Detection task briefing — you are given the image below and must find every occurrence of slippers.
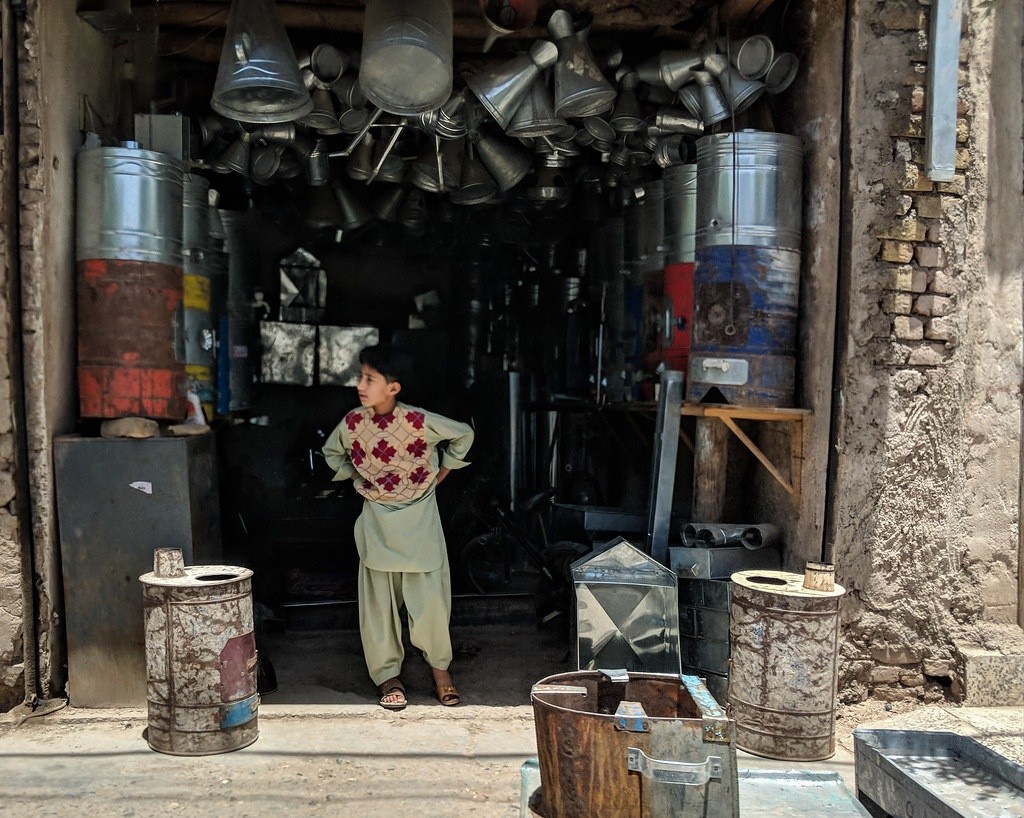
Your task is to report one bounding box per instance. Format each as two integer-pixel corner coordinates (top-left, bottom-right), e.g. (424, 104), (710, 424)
(433, 685), (460, 706)
(379, 678), (407, 708)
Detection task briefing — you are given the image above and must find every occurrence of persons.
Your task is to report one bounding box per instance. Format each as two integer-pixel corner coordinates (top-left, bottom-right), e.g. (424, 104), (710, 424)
(323, 344), (475, 708)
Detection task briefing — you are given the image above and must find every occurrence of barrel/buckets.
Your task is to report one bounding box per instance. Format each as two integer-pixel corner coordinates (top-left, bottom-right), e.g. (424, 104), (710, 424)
(73, 0), (803, 421)
(140, 547), (260, 755)
(728, 561), (845, 761)
